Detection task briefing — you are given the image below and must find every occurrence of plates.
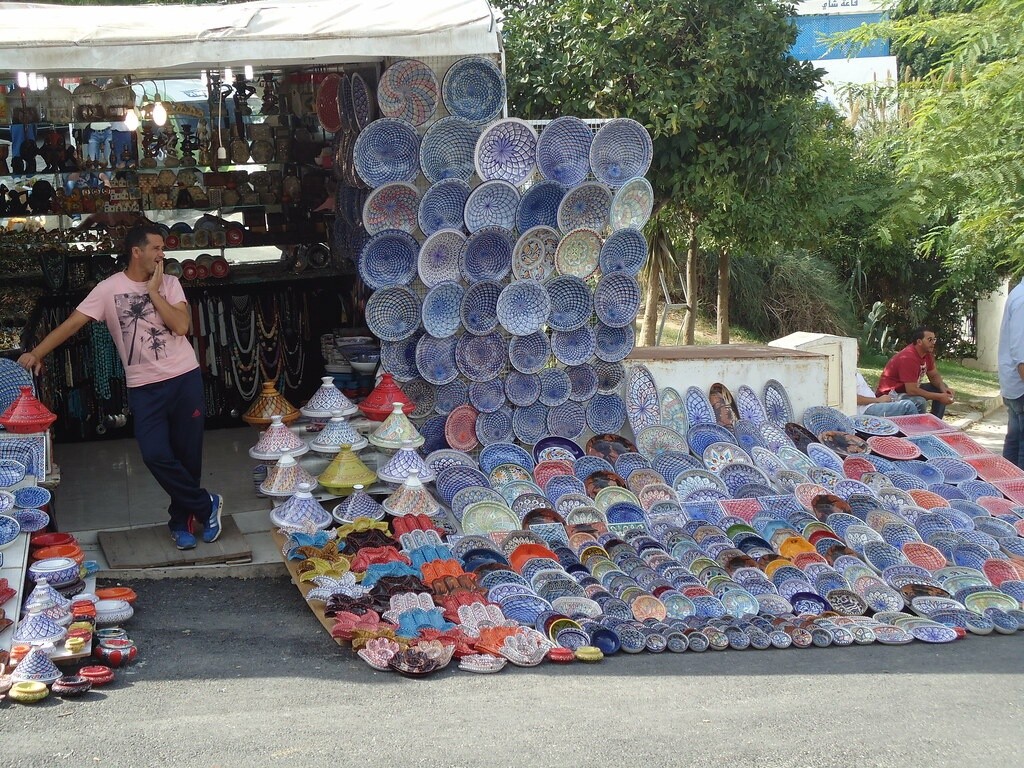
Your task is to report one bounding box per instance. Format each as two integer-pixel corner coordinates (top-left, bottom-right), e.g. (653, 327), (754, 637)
(0, 358), (49, 549)
(158, 216), (243, 281)
(314, 56), (1024, 661)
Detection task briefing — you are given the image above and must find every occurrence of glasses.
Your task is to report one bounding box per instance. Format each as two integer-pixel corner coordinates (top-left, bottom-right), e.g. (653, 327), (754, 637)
(922, 338), (938, 343)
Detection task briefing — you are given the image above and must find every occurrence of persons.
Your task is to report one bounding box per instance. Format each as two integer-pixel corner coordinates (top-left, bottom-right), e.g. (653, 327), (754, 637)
(17, 223), (224, 551)
(857, 344), (918, 415)
(876, 330), (954, 421)
(1000, 274), (1024, 473)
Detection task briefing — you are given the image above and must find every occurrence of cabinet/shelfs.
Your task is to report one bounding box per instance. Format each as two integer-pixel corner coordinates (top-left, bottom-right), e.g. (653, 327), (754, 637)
(0, 61), (382, 298)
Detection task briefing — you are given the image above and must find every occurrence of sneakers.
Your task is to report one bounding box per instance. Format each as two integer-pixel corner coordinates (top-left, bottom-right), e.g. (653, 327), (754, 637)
(171, 526), (196, 550)
(202, 494), (223, 543)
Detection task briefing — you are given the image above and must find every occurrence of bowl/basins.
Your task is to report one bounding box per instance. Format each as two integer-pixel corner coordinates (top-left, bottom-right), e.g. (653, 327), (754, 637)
(0, 534), (139, 702)
